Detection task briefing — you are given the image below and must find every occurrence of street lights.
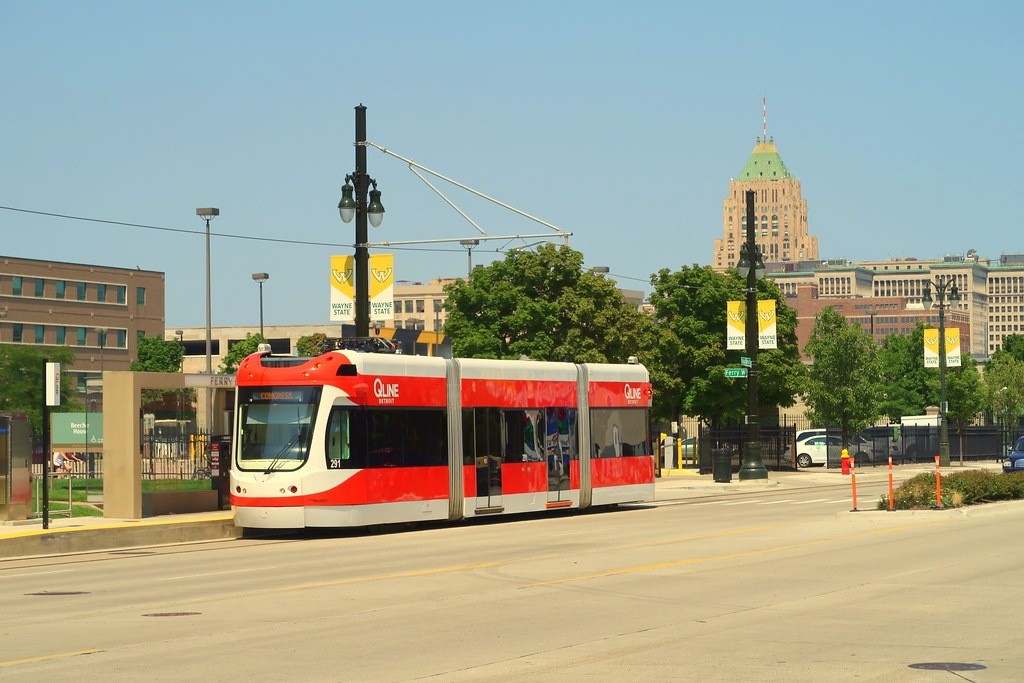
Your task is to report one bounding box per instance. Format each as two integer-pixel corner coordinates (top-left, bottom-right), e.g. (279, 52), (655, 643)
(338, 171), (386, 339)
(460, 239), (479, 286)
(736, 241), (768, 479)
(253, 273), (269, 343)
(923, 278), (960, 467)
(196, 207), (219, 442)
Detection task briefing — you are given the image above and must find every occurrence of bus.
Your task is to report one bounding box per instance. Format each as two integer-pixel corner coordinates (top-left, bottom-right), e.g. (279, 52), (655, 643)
(229, 344), (661, 536)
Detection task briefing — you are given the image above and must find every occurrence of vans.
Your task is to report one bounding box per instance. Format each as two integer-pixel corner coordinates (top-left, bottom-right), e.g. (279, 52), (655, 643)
(796, 429), (879, 454)
(858, 425), (935, 465)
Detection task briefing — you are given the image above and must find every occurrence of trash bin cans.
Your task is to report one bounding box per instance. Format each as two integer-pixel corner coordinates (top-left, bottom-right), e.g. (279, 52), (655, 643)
(711, 449), (732, 483)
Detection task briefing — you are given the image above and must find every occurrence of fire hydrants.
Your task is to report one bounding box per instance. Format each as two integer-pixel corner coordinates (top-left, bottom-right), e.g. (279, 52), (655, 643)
(841, 448), (850, 475)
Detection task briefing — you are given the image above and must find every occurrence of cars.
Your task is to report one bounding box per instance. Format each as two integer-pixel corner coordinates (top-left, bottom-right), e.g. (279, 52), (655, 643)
(681, 438), (698, 459)
(1003, 435), (1024, 472)
(783, 435), (875, 468)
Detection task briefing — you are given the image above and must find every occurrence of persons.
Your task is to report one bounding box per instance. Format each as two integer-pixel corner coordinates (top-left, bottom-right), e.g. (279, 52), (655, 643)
(53, 452), (81, 479)
